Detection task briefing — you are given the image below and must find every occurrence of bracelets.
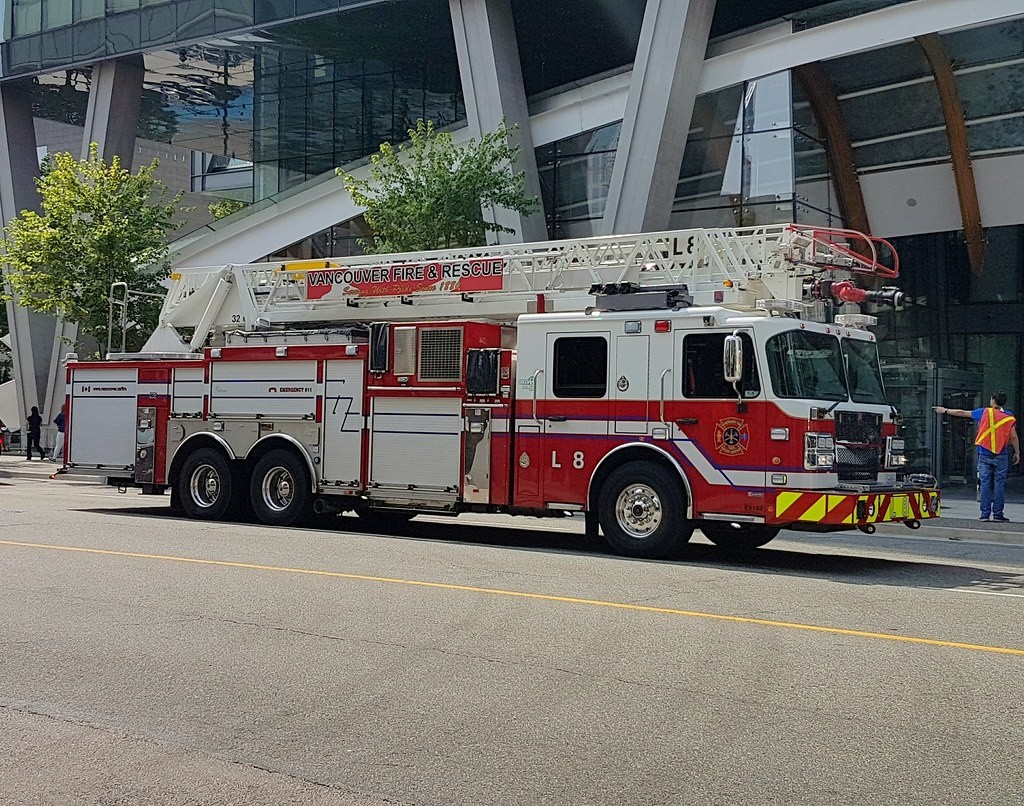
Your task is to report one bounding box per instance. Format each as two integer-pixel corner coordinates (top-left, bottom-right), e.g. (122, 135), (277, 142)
(945, 411), (947, 413)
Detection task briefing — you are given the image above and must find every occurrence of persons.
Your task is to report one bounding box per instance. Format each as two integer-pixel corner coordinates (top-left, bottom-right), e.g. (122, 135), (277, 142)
(24, 406), (45, 460)
(49, 403), (65, 462)
(931, 390), (1020, 523)
(0, 420), (12, 452)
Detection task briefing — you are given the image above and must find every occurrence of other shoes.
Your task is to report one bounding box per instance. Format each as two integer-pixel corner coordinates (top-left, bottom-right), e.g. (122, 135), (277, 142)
(49, 457), (56, 462)
(40, 454), (46, 460)
(26, 457), (31, 460)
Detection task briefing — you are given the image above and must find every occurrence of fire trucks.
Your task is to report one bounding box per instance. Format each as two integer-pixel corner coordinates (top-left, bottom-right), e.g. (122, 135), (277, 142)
(49, 224), (940, 559)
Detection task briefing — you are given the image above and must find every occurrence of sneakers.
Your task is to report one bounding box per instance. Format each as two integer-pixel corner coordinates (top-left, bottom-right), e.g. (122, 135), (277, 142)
(978, 515), (990, 521)
(993, 515), (1010, 522)
